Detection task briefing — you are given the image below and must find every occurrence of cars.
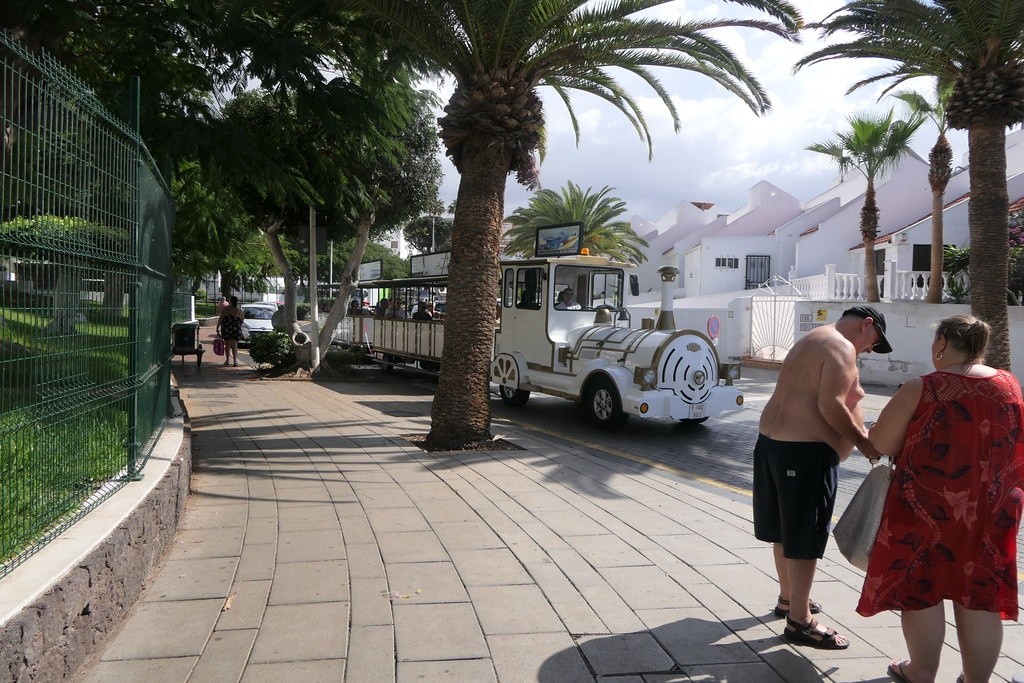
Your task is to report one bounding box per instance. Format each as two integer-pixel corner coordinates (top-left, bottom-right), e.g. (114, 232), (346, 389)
(236, 303), (279, 348)
(254, 300), (284, 314)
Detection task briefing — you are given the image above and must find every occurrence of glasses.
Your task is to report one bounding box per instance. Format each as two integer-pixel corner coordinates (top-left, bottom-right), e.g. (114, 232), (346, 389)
(873, 341), (881, 348)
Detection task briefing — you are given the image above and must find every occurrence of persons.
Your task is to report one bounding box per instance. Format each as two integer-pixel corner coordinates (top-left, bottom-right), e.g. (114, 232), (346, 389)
(411, 301), (440, 320)
(321, 303), (331, 311)
(216, 297), (230, 315)
(385, 298), (405, 319)
(854, 315), (1024, 683)
(555, 288), (581, 309)
(374, 298), (389, 318)
(272, 305), (286, 331)
(518, 291), (525, 307)
(216, 296), (245, 367)
(346, 300), (361, 317)
(752, 305), (893, 649)
(362, 299), (371, 315)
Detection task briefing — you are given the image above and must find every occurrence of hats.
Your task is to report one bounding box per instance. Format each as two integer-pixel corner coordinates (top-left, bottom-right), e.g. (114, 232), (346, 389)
(842, 305), (893, 353)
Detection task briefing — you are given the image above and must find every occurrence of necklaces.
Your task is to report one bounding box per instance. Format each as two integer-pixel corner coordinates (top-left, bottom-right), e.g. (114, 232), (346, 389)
(939, 362), (982, 370)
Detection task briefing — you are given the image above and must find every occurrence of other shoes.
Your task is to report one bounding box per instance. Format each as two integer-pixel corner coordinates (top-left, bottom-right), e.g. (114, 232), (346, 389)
(233, 363), (238, 366)
(224, 361), (229, 365)
(887, 658), (912, 683)
(956, 671), (964, 683)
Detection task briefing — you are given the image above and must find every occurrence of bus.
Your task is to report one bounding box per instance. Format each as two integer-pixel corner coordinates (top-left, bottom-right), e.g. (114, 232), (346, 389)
(317, 221), (746, 433)
(80, 278), (130, 308)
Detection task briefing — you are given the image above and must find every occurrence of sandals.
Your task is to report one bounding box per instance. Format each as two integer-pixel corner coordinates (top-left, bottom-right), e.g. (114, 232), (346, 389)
(784, 614), (850, 649)
(774, 596), (822, 617)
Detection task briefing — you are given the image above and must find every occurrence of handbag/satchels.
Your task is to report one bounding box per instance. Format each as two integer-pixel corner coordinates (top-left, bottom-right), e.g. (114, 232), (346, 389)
(214, 333), (224, 356)
(832, 454), (895, 573)
